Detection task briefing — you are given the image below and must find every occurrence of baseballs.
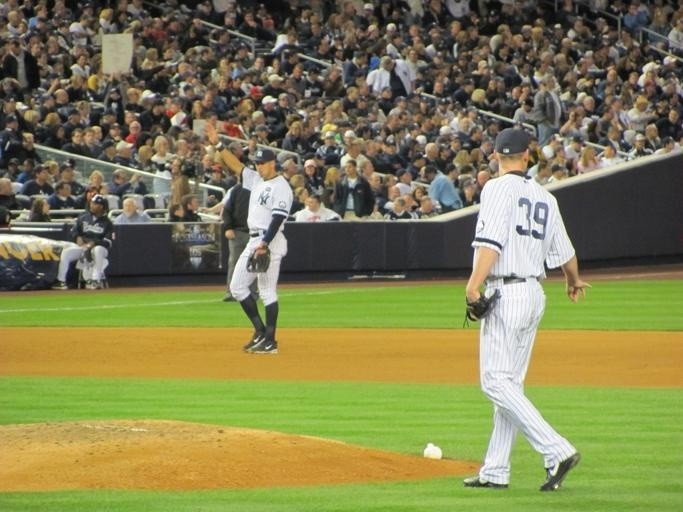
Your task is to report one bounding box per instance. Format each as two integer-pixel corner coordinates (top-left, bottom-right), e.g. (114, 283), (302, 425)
(485, 289), (495, 299)
(424, 446), (441, 459)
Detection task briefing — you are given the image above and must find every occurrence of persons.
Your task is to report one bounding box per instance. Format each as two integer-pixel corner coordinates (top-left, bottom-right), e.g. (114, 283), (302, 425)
(221, 182), (261, 304)
(462, 129), (593, 491)
(53, 194), (114, 290)
(204, 120), (293, 353)
(1, 1), (681, 229)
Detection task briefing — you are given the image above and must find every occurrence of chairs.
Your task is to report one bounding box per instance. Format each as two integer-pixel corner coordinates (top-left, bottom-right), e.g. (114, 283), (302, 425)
(0, 162), (172, 223)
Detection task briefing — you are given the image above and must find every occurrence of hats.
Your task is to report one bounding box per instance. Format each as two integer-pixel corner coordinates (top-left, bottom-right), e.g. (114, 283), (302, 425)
(2, 70), (83, 174)
(252, 148), (276, 163)
(90, 193), (106, 204)
(494, 127), (532, 155)
(141, 89), (156, 101)
(263, 66), (503, 169)
(140, 9), (152, 19)
(547, 56), (677, 143)
(363, 2), (397, 34)
(244, 161), (257, 171)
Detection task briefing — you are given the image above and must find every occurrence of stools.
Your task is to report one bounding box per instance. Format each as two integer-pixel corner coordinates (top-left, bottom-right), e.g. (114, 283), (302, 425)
(76, 255), (111, 291)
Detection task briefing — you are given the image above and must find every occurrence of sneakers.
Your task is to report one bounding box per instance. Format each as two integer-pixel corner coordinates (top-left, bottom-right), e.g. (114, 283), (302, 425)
(50, 280), (68, 290)
(242, 329), (266, 352)
(247, 339), (279, 355)
(222, 291), (237, 303)
(85, 279), (101, 290)
(537, 451), (581, 493)
(462, 476), (509, 490)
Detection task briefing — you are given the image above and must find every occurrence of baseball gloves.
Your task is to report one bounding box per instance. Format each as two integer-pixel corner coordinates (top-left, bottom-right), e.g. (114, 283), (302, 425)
(466, 290), (500, 321)
(247, 246), (270, 272)
